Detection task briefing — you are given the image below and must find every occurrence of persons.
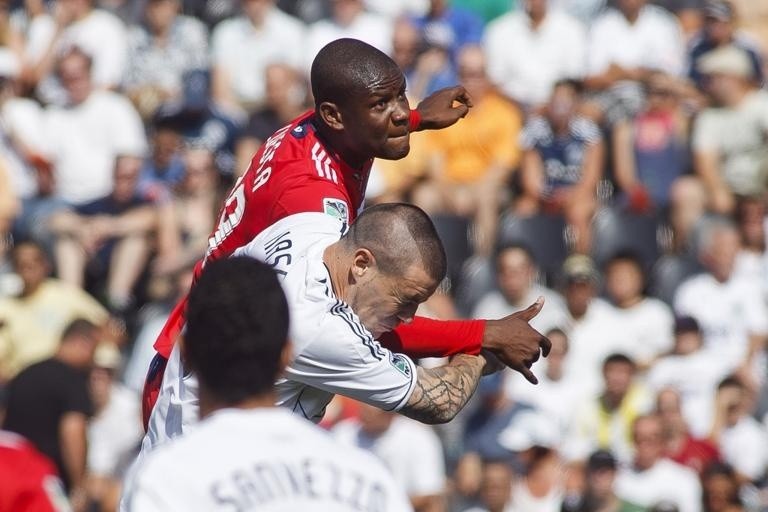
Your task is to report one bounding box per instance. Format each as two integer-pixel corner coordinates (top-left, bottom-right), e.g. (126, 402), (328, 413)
(2, 0), (768, 512)
